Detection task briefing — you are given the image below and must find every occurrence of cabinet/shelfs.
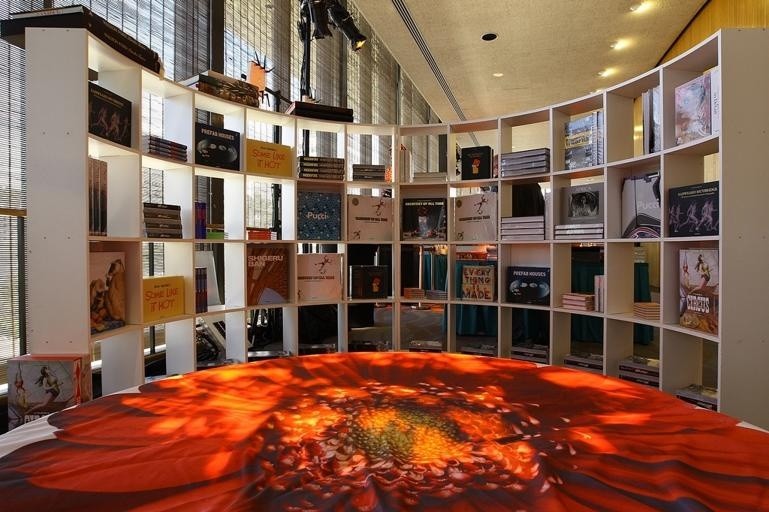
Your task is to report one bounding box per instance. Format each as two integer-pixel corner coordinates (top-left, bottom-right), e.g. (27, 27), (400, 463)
(24, 25), (769, 429)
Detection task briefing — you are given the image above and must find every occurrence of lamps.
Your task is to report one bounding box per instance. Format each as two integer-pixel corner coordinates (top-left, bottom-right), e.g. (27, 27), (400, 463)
(298, 0), (367, 103)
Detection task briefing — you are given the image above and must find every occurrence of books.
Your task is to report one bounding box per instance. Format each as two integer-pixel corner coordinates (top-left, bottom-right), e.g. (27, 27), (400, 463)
(347, 164), (392, 354)
(9, 4), (159, 63)
(89, 82), (132, 337)
(299, 155), (345, 356)
(139, 136), (185, 322)
(454, 146), (496, 357)
(555, 108), (604, 372)
(195, 108), (240, 371)
(1, 13), (160, 75)
(666, 65), (719, 410)
(500, 148), (550, 364)
(619, 86), (661, 387)
(399, 173), (447, 351)
(245, 138), (293, 363)
(178, 71), (354, 123)
